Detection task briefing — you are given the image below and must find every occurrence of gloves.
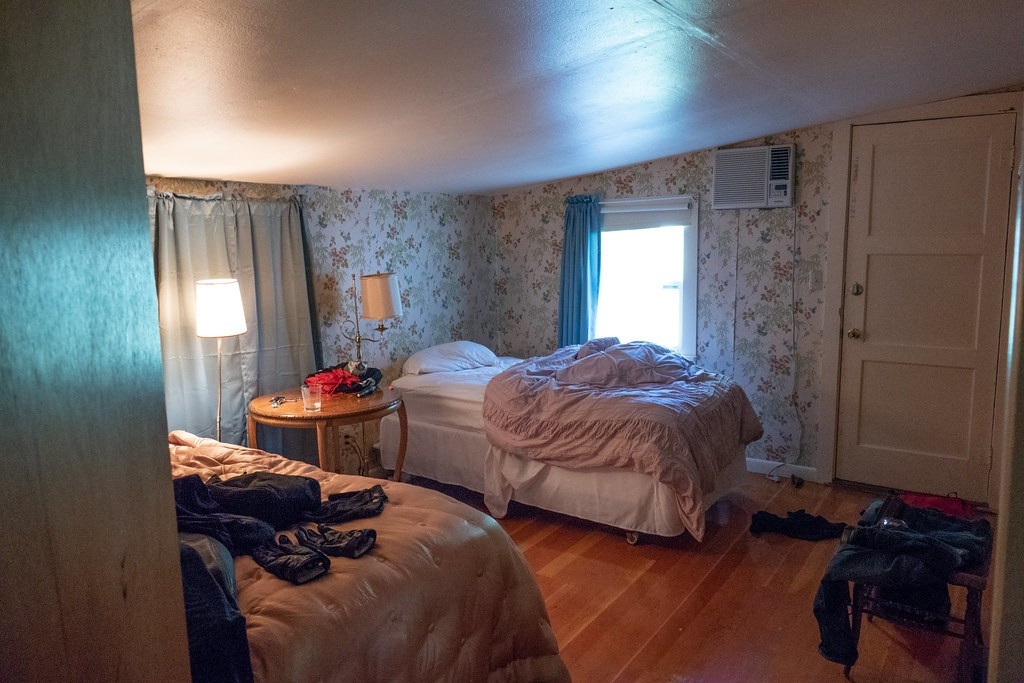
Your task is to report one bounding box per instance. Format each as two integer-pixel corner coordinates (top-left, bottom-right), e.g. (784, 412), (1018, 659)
(248, 532), (331, 586)
(304, 484), (388, 524)
(294, 523), (378, 562)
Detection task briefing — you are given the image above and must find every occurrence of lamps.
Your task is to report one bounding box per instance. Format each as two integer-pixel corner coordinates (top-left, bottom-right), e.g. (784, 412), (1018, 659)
(340, 273), (402, 476)
(195, 278), (248, 442)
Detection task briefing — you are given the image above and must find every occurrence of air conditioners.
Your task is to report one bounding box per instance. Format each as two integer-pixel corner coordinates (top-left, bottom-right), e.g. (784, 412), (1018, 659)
(712, 143), (794, 211)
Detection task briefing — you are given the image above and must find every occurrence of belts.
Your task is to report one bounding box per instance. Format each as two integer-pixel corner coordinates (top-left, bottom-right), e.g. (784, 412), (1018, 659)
(840, 495), (906, 544)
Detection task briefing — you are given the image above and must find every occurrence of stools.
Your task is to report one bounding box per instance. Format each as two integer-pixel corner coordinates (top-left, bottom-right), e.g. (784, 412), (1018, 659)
(843, 485), (988, 683)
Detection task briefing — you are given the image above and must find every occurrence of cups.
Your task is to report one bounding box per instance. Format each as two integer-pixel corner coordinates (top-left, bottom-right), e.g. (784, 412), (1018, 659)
(301, 384), (323, 412)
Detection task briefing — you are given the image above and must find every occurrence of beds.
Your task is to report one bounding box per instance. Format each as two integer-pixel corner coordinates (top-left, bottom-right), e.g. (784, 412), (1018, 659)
(168, 430), (572, 683)
(379, 355), (763, 545)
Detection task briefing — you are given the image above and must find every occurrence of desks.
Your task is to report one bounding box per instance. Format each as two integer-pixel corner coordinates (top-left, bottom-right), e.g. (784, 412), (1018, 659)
(248, 386), (409, 481)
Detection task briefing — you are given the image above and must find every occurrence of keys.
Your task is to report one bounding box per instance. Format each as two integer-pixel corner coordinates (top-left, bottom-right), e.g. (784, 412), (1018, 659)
(270, 396), (297, 407)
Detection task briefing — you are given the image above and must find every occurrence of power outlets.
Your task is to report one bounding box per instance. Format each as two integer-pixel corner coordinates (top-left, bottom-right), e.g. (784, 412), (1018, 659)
(342, 429), (351, 448)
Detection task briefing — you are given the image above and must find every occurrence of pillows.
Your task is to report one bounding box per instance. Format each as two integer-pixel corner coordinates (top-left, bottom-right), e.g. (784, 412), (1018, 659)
(401, 342), (503, 374)
